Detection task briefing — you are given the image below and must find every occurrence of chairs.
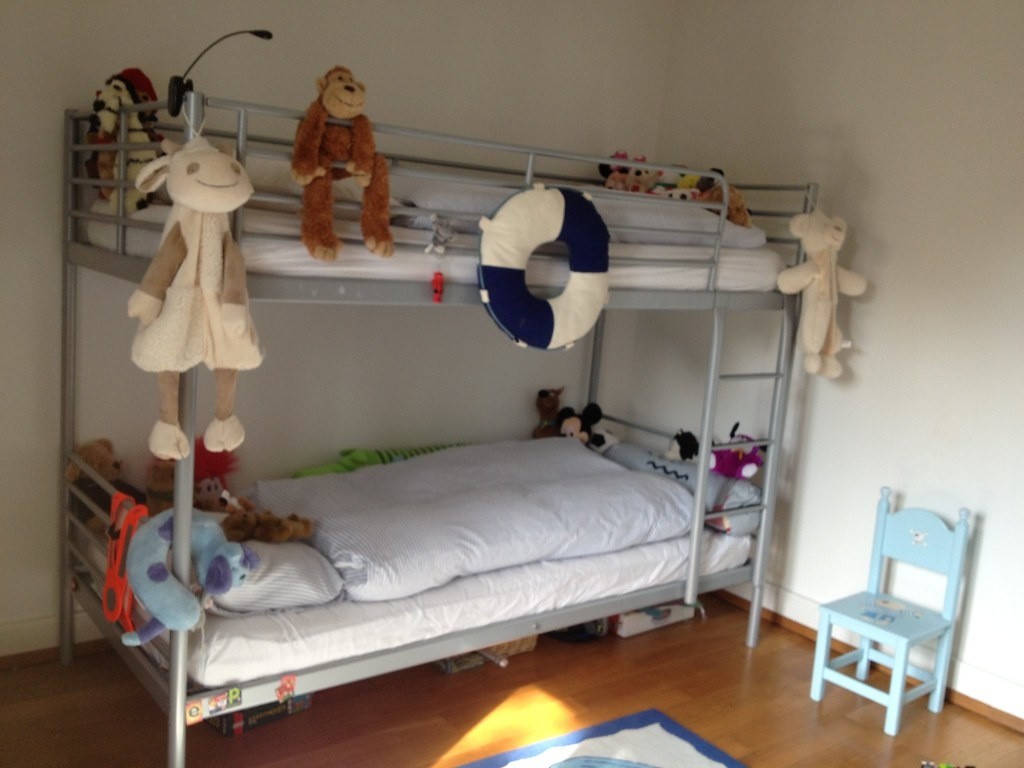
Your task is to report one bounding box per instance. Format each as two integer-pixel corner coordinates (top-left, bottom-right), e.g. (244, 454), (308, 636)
(808, 486), (970, 737)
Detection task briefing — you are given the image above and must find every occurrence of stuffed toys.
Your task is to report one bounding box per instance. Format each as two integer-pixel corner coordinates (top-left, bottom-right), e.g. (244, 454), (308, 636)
(776, 209), (867, 377)
(599, 148), (753, 227)
(664, 431), (717, 465)
(710, 422), (770, 479)
(143, 448), (176, 519)
(220, 499), (314, 542)
(532, 385), (565, 438)
(64, 438), (147, 538)
(121, 505), (260, 647)
(287, 65), (395, 261)
(124, 133), (263, 460)
(91, 68), (165, 216)
(289, 440), (497, 477)
(192, 433), (255, 513)
(556, 403), (602, 447)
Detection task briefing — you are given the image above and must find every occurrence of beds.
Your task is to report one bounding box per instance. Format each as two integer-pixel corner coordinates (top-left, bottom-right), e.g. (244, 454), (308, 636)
(57, 88), (821, 768)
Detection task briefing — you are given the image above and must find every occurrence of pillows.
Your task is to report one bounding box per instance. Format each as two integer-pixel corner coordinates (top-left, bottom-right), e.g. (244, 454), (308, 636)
(603, 442), (763, 536)
(198, 507), (345, 611)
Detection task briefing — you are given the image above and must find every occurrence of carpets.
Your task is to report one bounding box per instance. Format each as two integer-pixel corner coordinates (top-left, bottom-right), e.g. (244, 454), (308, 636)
(454, 709), (747, 768)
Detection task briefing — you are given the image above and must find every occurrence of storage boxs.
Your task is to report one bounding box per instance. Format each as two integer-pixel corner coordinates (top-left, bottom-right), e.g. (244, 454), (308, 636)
(608, 603), (695, 638)
(204, 694), (314, 738)
